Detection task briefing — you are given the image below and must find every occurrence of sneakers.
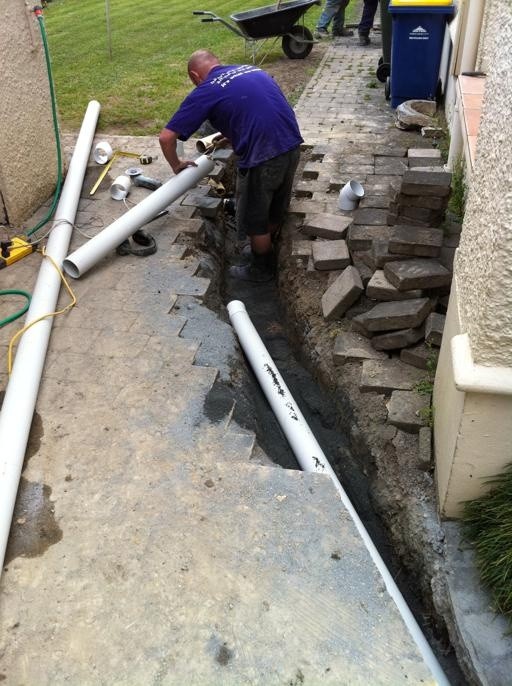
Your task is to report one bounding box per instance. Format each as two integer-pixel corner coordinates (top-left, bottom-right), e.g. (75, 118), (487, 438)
(230, 263), (273, 282)
(243, 244), (254, 260)
(314, 29), (330, 38)
(333, 29), (353, 36)
(359, 36), (371, 46)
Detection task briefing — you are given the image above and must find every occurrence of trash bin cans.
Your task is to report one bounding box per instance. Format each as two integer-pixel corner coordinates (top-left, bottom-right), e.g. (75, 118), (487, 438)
(376, 0), (455, 108)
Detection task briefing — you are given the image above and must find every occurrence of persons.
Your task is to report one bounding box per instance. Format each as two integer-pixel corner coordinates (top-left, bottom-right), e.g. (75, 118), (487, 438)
(357, 0), (380, 46)
(159, 50), (304, 281)
(314, 0), (353, 38)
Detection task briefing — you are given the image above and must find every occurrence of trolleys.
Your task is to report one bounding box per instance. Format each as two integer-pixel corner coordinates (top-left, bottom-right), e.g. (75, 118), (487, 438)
(192, 0), (322, 66)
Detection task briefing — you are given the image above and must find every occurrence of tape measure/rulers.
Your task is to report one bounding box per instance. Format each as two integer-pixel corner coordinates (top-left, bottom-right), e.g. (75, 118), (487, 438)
(89, 151), (152, 195)
